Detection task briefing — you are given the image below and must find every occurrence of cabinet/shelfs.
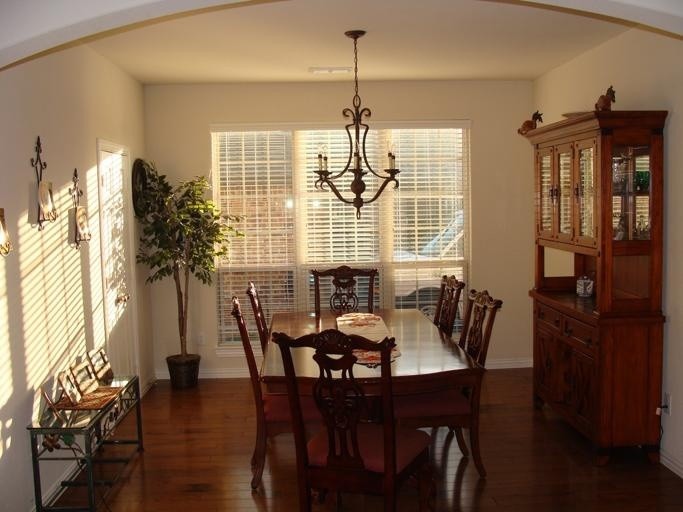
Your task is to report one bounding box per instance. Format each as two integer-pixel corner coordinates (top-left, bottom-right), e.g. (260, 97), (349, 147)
(520, 108), (670, 452)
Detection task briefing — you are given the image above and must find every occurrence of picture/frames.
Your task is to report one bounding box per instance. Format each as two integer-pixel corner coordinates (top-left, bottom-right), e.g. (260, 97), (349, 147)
(57, 370), (81, 405)
(88, 346), (112, 381)
(70, 360), (99, 395)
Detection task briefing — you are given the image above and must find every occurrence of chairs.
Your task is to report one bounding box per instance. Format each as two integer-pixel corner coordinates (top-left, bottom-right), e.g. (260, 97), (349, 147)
(432, 272), (465, 336)
(310, 263), (376, 311)
(395, 287), (503, 480)
(248, 280), (276, 358)
(231, 294), (337, 490)
(268, 326), (436, 511)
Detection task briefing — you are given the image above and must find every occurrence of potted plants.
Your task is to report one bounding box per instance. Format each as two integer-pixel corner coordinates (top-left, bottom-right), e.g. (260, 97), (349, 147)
(133, 158), (247, 388)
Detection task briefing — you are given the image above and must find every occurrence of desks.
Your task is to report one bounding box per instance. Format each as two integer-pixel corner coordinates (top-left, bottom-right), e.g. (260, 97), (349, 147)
(255, 308), (487, 512)
(25, 375), (148, 512)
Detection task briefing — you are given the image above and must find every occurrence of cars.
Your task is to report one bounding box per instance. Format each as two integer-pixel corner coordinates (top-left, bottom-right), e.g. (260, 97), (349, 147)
(421, 208), (465, 259)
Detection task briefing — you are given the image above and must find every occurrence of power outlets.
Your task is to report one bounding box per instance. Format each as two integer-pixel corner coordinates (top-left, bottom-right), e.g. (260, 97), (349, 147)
(663, 392), (671, 416)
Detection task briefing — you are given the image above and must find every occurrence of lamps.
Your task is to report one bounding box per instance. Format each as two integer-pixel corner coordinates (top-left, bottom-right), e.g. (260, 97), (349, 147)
(312, 28), (400, 220)
